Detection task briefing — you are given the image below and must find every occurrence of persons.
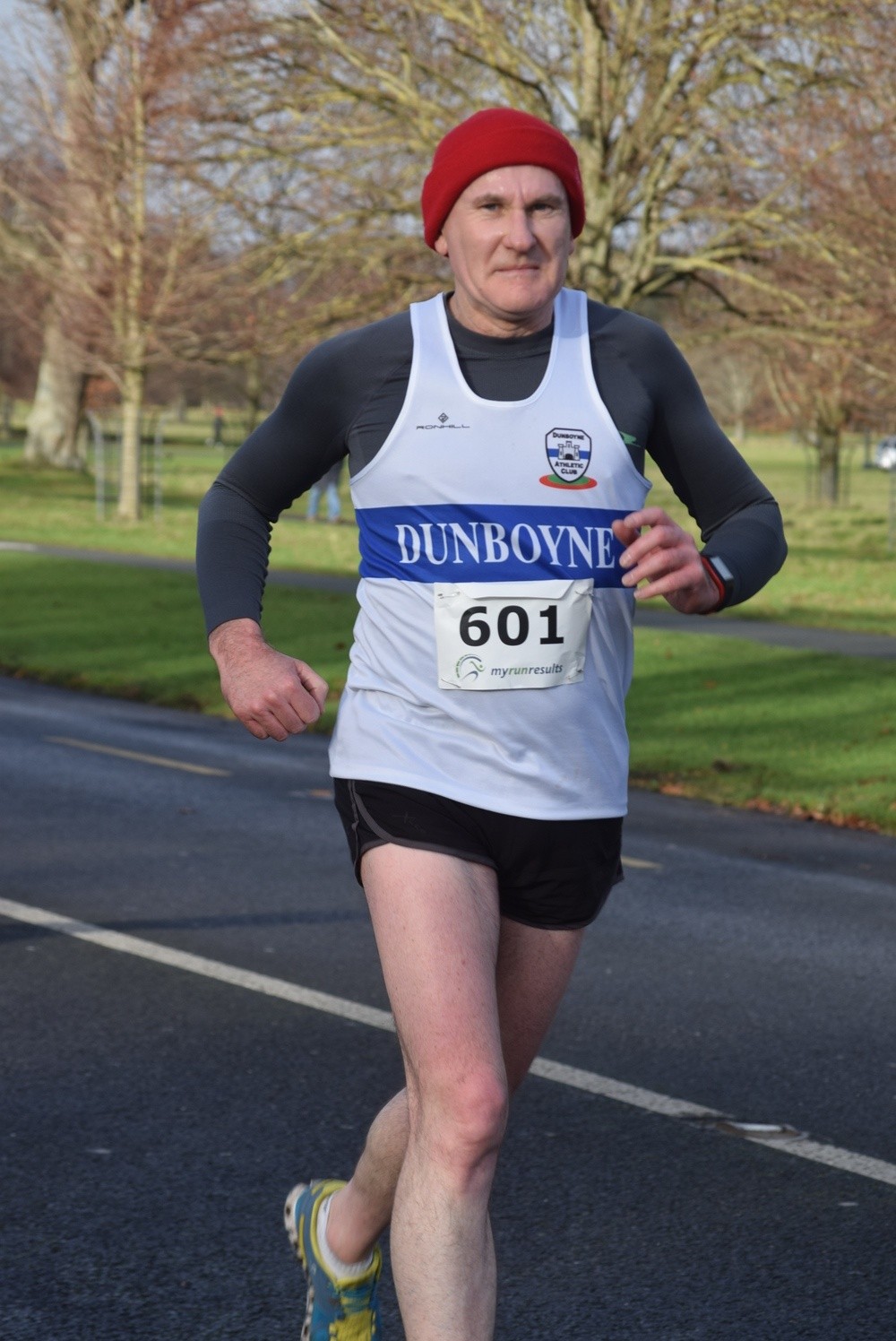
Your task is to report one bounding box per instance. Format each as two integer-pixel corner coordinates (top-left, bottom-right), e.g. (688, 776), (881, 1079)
(195, 106), (790, 1341)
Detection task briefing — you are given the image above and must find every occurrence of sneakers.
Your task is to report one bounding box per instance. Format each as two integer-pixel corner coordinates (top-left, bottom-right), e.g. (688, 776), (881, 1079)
(284, 1177), (383, 1341)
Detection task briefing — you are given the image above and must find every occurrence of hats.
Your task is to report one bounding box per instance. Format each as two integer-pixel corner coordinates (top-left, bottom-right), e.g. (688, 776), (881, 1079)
(421, 108), (586, 257)
